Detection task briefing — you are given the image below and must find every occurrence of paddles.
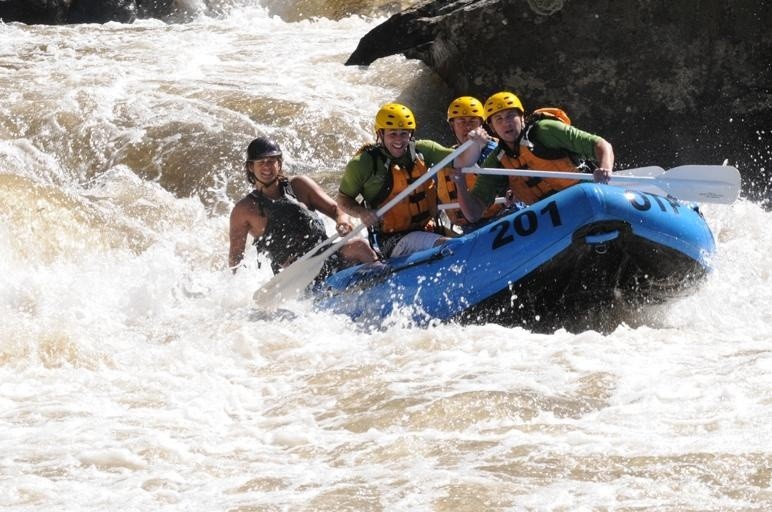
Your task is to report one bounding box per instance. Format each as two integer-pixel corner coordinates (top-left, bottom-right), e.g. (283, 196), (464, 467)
(461, 165), (741, 206)
(608, 167), (669, 202)
(252, 141), (472, 312)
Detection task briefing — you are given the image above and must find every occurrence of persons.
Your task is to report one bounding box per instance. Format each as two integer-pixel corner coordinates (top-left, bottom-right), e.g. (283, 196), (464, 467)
(333, 103), (489, 262)
(423, 94), (524, 234)
(227, 135), (380, 275)
(444, 90), (617, 226)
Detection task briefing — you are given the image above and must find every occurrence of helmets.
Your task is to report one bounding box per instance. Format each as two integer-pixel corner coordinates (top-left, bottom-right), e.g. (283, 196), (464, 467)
(375, 103), (416, 137)
(447, 96), (484, 123)
(247, 137), (282, 162)
(484, 91), (524, 122)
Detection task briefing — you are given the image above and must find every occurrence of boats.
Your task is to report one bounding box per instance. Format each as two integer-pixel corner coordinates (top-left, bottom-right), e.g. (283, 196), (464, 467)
(313, 182), (716, 335)
